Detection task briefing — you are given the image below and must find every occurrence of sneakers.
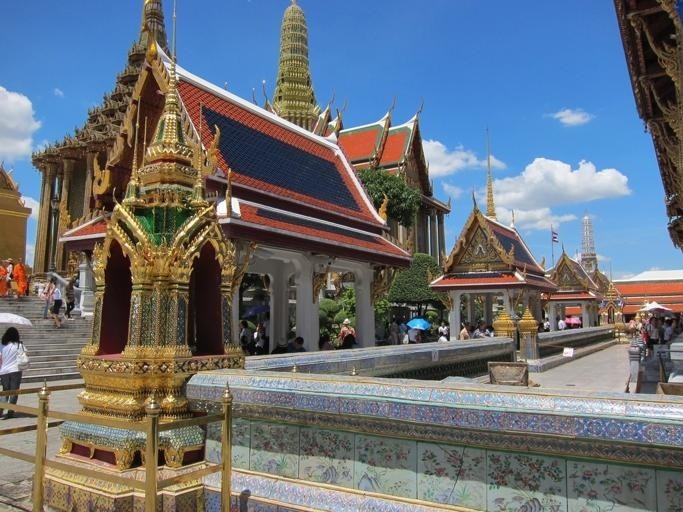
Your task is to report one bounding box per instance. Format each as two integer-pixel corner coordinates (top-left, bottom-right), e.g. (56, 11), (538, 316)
(62, 312), (76, 321)
(0, 413), (20, 420)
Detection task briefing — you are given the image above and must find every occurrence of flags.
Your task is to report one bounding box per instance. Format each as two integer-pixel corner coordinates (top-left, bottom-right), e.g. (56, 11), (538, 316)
(551, 230), (559, 242)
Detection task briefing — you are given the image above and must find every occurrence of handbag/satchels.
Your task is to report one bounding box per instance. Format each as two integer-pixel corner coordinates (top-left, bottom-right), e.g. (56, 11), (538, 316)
(16, 341), (30, 371)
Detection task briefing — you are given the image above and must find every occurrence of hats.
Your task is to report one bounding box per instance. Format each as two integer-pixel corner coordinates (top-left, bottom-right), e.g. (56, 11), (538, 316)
(343, 319), (351, 325)
(1, 257), (24, 263)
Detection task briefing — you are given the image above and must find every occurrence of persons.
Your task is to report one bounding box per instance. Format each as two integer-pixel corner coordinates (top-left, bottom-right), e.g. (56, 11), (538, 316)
(0, 326), (25, 419)
(0, 256), (78, 330)
